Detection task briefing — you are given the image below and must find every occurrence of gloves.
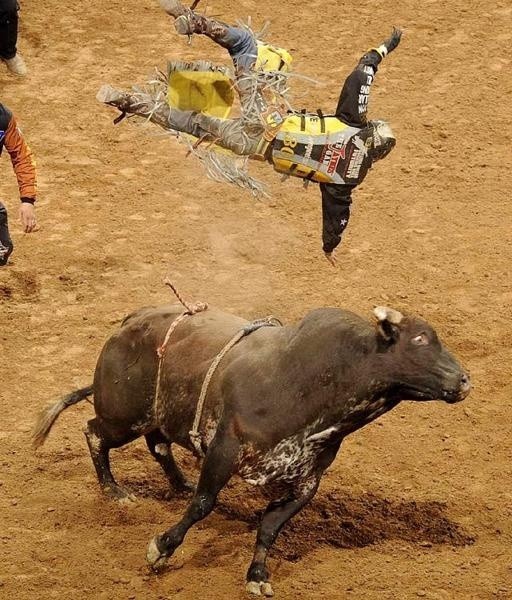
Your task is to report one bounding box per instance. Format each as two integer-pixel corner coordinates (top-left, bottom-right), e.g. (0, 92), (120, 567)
(380, 25), (403, 59)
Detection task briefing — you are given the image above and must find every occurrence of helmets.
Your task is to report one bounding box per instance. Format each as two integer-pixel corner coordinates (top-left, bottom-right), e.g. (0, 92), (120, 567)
(370, 120), (396, 162)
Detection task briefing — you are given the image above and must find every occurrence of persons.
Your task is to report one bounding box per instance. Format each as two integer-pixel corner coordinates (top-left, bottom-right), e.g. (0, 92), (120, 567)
(0, 0), (28, 77)
(96, 0), (404, 266)
(0, 102), (38, 266)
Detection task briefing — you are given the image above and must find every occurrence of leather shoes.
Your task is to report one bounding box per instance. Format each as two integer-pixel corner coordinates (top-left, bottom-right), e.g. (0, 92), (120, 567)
(95, 84), (124, 106)
(3, 51), (27, 75)
(162, 1), (195, 35)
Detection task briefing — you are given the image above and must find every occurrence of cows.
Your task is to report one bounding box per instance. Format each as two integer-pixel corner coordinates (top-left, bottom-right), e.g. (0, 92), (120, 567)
(29, 306), (472, 597)
